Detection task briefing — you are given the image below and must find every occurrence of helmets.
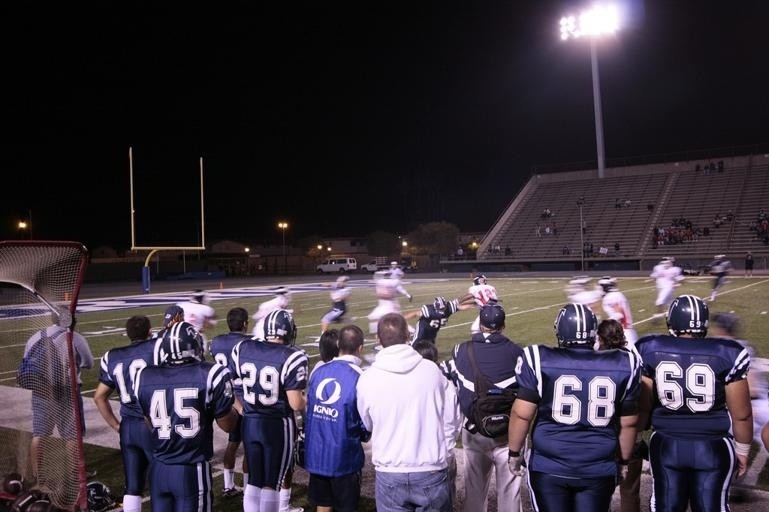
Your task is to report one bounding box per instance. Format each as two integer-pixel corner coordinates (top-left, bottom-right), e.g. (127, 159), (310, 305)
(552, 303), (598, 348)
(473, 275), (487, 285)
(665, 294), (709, 337)
(433, 295), (446, 315)
(264, 310), (297, 346)
(599, 276), (618, 292)
(164, 321), (203, 364)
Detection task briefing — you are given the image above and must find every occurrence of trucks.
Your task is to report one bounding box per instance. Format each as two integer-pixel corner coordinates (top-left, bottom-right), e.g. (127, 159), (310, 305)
(361, 257), (402, 275)
(316, 258), (357, 274)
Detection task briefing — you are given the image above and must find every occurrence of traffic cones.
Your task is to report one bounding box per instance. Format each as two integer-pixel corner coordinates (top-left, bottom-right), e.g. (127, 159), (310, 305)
(216, 280), (225, 289)
(62, 291), (70, 302)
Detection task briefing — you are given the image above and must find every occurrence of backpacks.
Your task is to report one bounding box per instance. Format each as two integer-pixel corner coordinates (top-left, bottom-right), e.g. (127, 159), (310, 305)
(16, 328), (67, 403)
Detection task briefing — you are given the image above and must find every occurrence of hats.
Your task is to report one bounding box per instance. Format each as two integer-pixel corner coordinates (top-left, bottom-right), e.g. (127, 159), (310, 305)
(164, 305), (183, 326)
(480, 302), (506, 331)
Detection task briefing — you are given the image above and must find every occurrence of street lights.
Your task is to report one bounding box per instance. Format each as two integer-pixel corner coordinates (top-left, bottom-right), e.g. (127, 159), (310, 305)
(560, 4), (623, 180)
(575, 195), (586, 273)
(17, 210), (34, 240)
(277, 220), (289, 274)
(317, 240), (332, 258)
(244, 247), (253, 277)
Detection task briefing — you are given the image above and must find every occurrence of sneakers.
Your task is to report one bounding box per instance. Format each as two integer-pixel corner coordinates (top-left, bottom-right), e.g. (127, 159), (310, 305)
(221, 484), (243, 498)
(280, 506), (304, 512)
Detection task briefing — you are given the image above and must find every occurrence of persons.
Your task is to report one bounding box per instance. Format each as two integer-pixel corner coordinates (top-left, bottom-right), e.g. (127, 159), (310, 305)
(368, 272), (413, 338)
(713, 215), (721, 228)
(726, 210), (734, 222)
(461, 276), (499, 334)
(643, 261), (686, 324)
(707, 313), (768, 501)
(535, 222), (556, 237)
(303, 325), (373, 512)
(507, 303), (642, 512)
(584, 241), (605, 257)
(749, 209), (769, 246)
(495, 244), (500, 255)
(542, 209), (551, 218)
(576, 194), (585, 207)
(356, 312), (466, 512)
(616, 198), (621, 208)
(582, 219), (586, 233)
(563, 247), (569, 254)
(744, 251), (755, 276)
(156, 306), (184, 338)
(376, 261), (412, 303)
(596, 319), (640, 511)
(94, 315), (163, 512)
(652, 215), (699, 248)
(138, 321), (244, 512)
(647, 201), (654, 211)
(414, 340), (439, 362)
(405, 296), (473, 343)
(228, 309), (309, 512)
(309, 329), (371, 376)
(315, 275), (358, 344)
(505, 245), (511, 255)
(458, 246), (463, 260)
(694, 160), (724, 174)
(212, 307), (254, 493)
(177, 290), (217, 354)
(488, 244), (492, 253)
(624, 198), (631, 207)
(23, 304), (95, 483)
(703, 254), (731, 301)
(614, 243), (620, 257)
(253, 286), (290, 337)
(631, 294), (751, 512)
(440, 302), (527, 512)
(598, 276), (638, 349)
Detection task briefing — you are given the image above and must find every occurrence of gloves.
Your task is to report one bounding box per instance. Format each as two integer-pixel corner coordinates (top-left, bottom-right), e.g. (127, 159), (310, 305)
(507, 450), (527, 477)
(618, 463), (629, 484)
(735, 442), (751, 480)
